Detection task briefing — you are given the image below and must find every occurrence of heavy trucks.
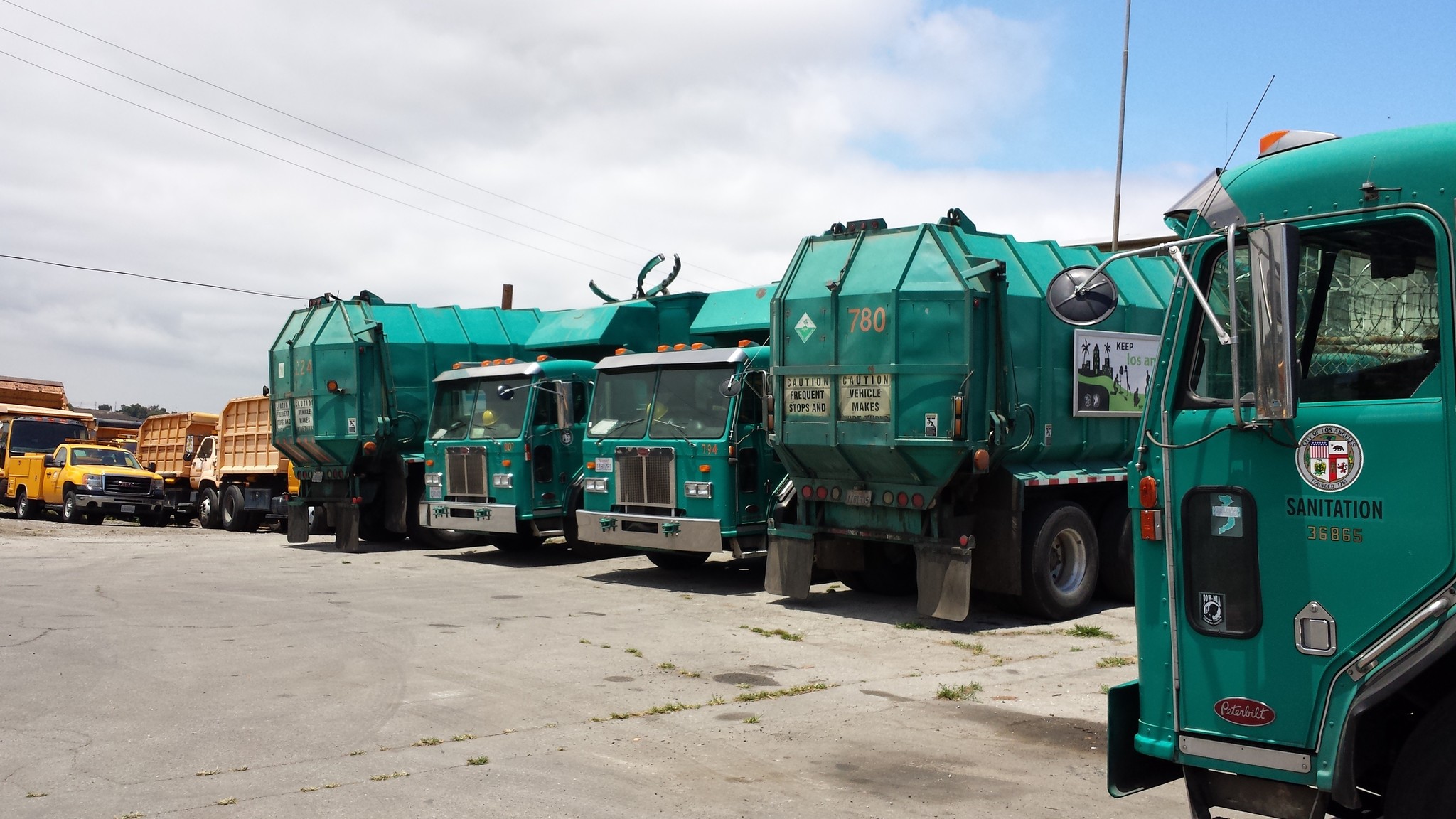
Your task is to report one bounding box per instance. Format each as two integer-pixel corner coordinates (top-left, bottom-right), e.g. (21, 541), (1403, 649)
(761, 205), (1251, 628)
(264, 250), (782, 571)
(1042, 122), (1456, 818)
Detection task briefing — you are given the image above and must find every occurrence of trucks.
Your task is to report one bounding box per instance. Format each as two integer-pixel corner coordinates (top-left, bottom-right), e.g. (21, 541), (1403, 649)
(0, 376), (303, 533)
